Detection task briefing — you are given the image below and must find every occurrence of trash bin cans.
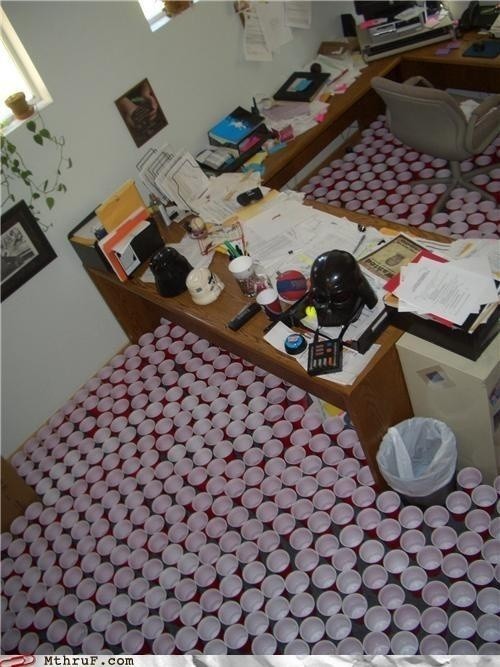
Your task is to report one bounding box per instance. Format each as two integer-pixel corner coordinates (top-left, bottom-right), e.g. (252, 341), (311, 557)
(375, 418), (457, 511)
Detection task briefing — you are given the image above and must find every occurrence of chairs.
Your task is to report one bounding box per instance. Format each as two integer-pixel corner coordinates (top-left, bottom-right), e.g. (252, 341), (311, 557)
(370, 75), (500, 215)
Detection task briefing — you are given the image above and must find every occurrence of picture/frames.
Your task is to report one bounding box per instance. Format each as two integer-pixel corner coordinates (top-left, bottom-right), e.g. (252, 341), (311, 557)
(0, 199), (58, 303)
(114, 77), (169, 148)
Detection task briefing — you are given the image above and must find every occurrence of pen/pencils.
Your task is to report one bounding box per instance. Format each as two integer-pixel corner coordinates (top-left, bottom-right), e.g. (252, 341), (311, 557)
(223, 239), (247, 261)
(327, 69), (348, 85)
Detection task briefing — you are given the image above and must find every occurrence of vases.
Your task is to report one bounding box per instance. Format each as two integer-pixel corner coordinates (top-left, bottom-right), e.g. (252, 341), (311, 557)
(5, 92), (29, 115)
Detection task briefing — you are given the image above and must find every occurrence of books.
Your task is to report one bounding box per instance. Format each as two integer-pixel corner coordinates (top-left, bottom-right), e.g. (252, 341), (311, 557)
(272, 71), (331, 103)
(208, 105), (266, 146)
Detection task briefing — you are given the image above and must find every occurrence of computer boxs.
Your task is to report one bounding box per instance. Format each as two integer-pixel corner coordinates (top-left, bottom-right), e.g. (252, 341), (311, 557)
(356, 10), (455, 62)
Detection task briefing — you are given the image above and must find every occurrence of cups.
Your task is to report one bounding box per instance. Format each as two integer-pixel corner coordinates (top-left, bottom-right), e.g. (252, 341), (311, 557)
(298, 112), (500, 241)
(255, 287), (283, 322)
(0, 316), (500, 654)
(227, 253), (259, 298)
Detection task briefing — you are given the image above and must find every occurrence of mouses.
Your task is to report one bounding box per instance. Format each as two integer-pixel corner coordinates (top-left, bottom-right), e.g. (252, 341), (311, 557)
(474, 40), (485, 52)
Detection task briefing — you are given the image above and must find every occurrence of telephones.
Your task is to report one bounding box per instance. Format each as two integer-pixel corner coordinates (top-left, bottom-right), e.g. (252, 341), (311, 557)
(461, 0), (500, 30)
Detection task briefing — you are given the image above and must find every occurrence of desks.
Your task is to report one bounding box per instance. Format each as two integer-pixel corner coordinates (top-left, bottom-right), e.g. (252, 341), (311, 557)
(84, 175), (459, 490)
(226, 30), (500, 186)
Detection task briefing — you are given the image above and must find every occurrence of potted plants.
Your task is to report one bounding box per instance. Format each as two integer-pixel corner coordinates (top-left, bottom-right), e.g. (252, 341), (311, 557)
(162, 0), (193, 17)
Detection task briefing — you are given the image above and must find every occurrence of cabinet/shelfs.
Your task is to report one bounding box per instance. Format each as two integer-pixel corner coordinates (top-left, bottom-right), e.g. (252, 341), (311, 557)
(394, 332), (499, 483)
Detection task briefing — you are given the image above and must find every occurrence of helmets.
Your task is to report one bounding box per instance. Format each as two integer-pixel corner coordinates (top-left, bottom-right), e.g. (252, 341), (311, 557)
(310, 249), (378, 326)
